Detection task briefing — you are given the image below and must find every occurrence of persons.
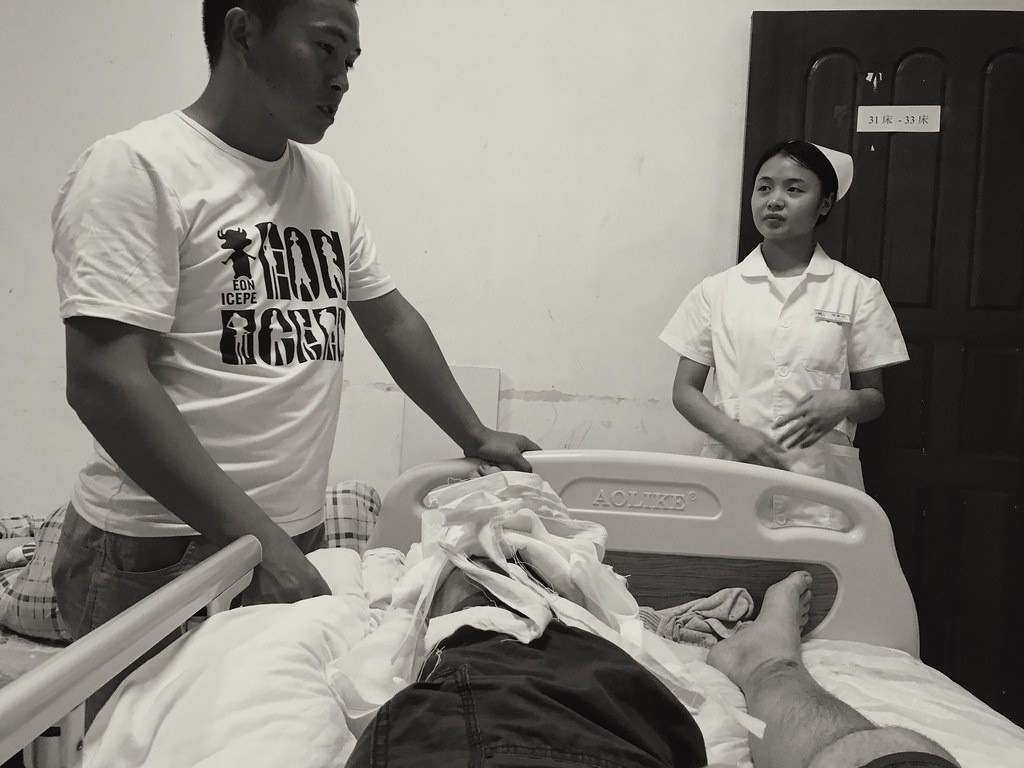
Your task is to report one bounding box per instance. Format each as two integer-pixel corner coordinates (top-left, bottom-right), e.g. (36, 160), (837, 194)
(655, 137), (910, 534)
(343, 460), (962, 768)
(50, 0), (543, 735)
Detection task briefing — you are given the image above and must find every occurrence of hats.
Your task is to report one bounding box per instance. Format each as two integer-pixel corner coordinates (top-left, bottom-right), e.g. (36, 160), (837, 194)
(808, 142), (854, 203)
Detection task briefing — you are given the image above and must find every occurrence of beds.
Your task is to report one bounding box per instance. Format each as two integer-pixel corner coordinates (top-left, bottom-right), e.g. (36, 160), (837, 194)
(0, 447), (1024, 768)
